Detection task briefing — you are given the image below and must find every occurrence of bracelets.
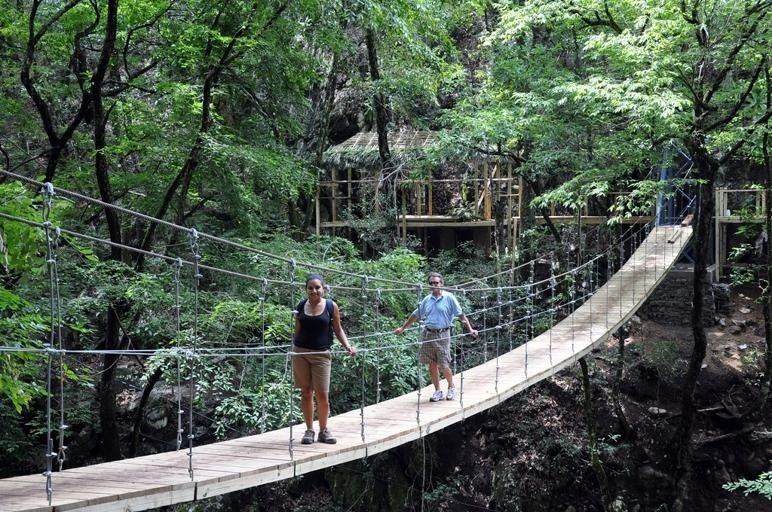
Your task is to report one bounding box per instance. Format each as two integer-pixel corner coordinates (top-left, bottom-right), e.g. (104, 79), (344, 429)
(344, 345), (352, 349)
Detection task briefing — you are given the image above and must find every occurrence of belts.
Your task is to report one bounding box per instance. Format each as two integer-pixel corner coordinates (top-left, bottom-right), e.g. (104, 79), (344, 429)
(425, 326), (451, 334)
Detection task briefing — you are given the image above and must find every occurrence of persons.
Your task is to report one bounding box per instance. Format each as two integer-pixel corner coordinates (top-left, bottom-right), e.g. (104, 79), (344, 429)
(291, 276), (358, 446)
(392, 272), (479, 404)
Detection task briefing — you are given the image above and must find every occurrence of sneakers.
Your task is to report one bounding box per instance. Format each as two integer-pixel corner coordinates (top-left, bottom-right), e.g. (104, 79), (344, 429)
(446, 386), (456, 400)
(318, 431), (337, 443)
(431, 391), (444, 402)
(301, 429), (314, 443)
